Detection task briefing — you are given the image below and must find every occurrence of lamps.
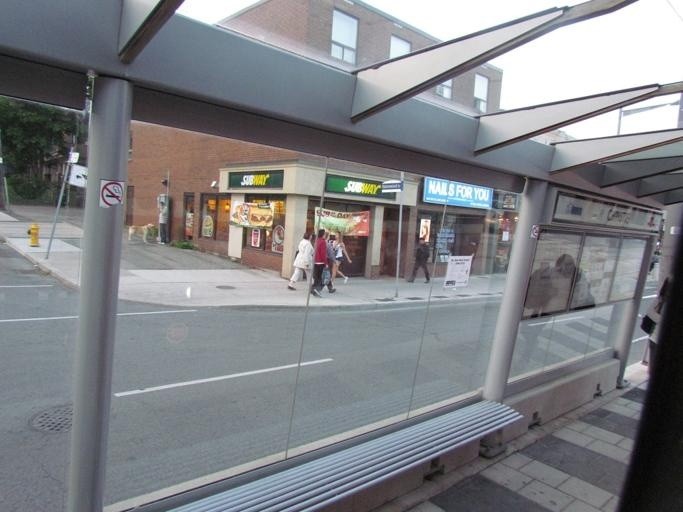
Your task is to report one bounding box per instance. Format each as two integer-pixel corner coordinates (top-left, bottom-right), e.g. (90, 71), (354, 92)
(225, 199), (230, 212)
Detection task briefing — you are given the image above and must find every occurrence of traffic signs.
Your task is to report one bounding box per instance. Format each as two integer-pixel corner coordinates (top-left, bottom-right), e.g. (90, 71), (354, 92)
(380, 182), (401, 189)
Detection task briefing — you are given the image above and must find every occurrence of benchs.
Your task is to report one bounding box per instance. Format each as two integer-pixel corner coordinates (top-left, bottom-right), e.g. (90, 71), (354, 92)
(163, 399), (524, 512)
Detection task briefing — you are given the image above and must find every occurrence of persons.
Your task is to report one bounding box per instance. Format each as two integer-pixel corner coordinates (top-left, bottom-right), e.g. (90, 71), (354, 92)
(310, 229), (328, 299)
(331, 231), (352, 284)
(644, 276), (671, 375)
(157, 200), (168, 246)
(287, 232), (314, 291)
(310, 231), (337, 293)
(404, 238), (430, 284)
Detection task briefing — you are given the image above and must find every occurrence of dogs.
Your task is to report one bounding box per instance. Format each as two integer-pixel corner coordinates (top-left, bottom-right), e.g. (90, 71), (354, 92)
(128, 224), (155, 244)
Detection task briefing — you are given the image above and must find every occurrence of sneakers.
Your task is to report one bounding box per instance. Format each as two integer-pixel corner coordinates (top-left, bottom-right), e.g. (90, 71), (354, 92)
(287, 284), (297, 291)
(310, 287), (337, 298)
(344, 276), (348, 284)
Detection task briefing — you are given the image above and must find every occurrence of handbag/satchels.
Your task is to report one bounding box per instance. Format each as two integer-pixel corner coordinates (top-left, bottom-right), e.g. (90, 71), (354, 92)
(321, 270), (330, 285)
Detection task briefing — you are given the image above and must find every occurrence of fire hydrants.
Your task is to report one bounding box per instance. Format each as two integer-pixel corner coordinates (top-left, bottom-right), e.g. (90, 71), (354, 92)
(28, 222), (39, 247)
